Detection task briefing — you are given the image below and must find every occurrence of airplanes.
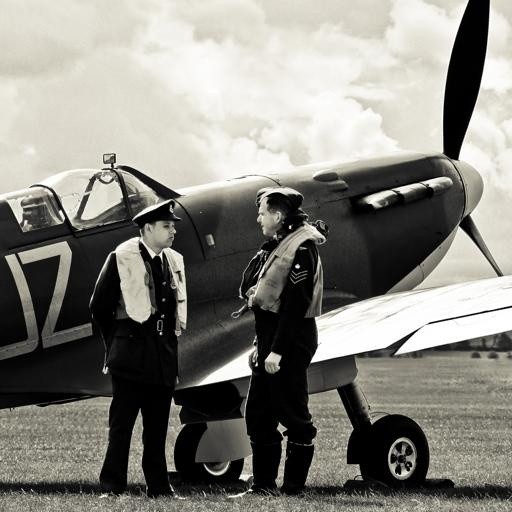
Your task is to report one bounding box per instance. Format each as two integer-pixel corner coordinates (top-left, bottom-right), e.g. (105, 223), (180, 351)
(0, 0), (512, 496)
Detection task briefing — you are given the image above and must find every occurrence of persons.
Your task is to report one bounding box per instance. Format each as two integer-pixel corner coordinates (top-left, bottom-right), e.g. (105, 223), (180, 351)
(19, 195), (56, 233)
(88, 195), (188, 498)
(225, 185), (327, 500)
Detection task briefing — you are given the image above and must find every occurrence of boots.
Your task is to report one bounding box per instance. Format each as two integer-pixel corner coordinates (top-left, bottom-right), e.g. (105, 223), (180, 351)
(226, 438), (283, 502)
(280, 441), (314, 495)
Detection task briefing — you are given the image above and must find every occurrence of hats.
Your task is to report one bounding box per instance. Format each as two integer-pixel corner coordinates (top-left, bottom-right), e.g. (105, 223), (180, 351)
(132, 199), (182, 229)
(256, 186), (303, 207)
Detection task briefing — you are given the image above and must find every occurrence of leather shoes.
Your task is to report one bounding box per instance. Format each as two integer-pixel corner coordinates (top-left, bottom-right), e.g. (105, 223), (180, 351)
(146, 484), (187, 501)
(100, 480), (127, 497)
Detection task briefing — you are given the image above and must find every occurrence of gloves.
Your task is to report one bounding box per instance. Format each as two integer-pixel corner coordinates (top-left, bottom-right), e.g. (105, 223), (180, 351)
(264, 351), (282, 375)
